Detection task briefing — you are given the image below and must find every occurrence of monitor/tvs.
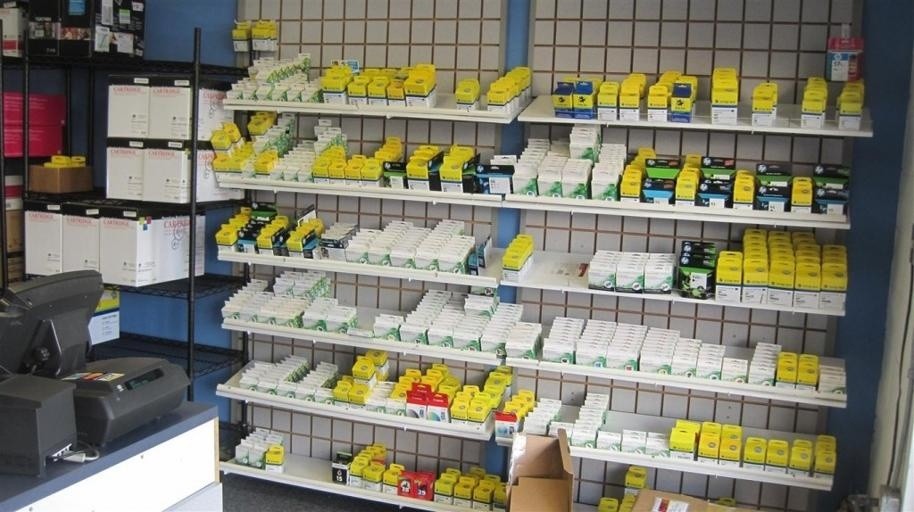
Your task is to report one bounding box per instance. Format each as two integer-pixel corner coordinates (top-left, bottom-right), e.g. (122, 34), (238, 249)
(1, 269), (105, 377)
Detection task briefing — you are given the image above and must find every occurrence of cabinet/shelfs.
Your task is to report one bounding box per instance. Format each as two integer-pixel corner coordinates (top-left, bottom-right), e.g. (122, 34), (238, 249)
(213, 99), (873, 511)
(0, 2), (253, 462)
